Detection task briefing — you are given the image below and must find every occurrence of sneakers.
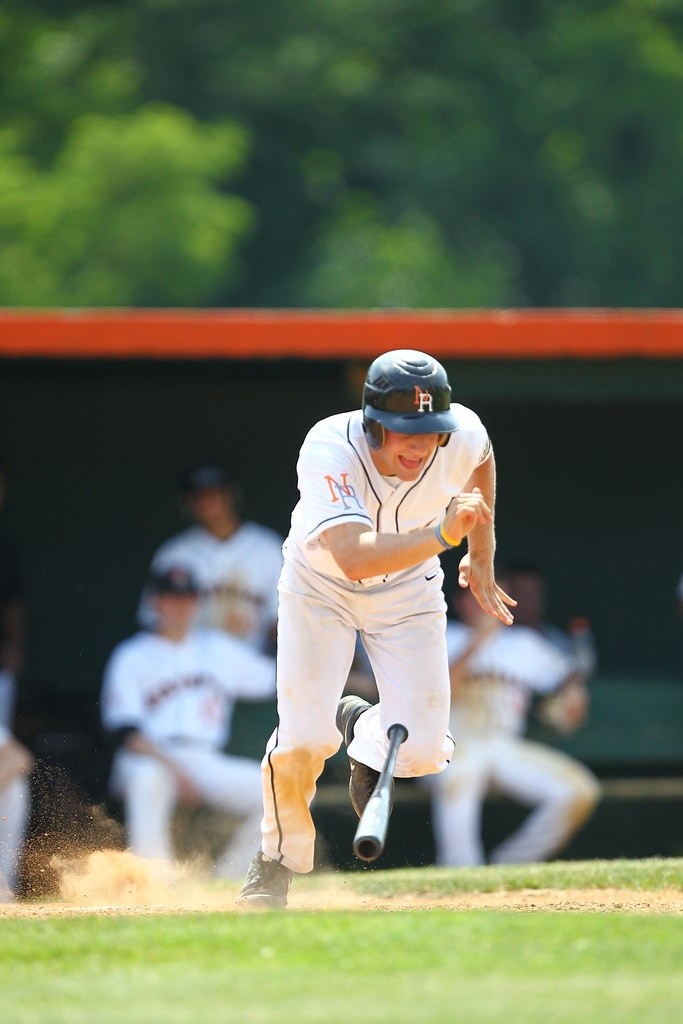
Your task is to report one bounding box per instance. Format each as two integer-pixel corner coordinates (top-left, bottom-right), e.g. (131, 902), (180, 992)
(336, 695), (395, 820)
(237, 851), (292, 909)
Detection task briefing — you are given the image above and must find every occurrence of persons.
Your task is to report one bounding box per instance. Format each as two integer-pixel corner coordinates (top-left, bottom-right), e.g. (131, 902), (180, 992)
(102, 467), (284, 883)
(429, 568), (605, 865)
(237, 348), (518, 911)
(0, 651), (37, 902)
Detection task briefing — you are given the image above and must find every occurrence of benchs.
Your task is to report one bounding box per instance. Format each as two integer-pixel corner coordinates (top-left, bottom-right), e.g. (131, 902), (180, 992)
(221, 675), (683, 811)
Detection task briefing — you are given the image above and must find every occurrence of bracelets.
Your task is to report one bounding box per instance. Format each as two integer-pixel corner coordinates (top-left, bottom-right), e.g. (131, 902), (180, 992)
(434, 521), (462, 552)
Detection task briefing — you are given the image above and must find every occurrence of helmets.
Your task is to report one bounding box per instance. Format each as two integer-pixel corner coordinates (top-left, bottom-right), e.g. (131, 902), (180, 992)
(362, 349), (459, 433)
(183, 465), (242, 499)
(157, 569), (197, 596)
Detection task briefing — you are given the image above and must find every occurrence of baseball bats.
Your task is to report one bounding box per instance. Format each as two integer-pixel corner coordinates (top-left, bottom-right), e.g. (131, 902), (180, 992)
(352, 722), (410, 862)
